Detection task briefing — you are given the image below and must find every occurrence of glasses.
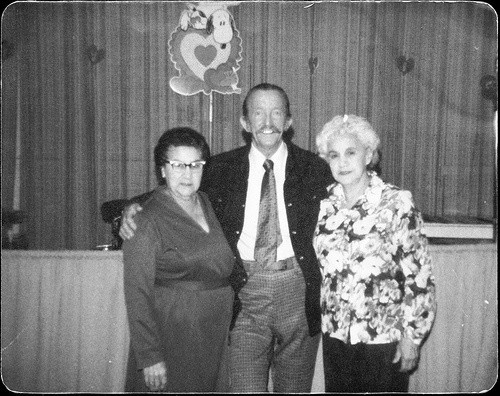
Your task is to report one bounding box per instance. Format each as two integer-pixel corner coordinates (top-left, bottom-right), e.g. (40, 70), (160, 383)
(164, 159), (206, 171)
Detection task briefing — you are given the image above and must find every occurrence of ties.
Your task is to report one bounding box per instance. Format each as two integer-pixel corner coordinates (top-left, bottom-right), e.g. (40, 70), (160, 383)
(254, 158), (283, 269)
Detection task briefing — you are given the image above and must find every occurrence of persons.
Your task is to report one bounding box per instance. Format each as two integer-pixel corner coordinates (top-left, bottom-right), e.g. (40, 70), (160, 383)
(119, 126), (236, 396)
(313, 112), (437, 396)
(113, 82), (335, 396)
